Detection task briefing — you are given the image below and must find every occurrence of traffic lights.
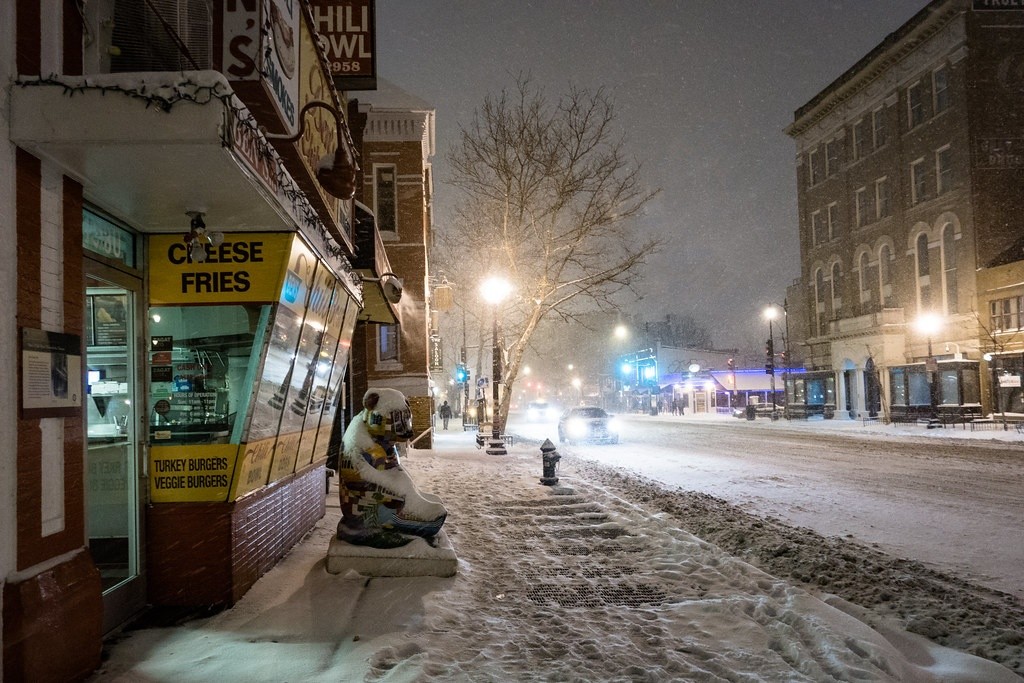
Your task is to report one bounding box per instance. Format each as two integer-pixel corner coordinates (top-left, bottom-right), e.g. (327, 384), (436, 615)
(457, 366), (466, 382)
(766, 338), (773, 356)
(467, 368), (471, 381)
(481, 278), (508, 456)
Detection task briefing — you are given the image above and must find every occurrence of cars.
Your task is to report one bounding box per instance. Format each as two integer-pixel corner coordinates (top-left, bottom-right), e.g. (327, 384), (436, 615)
(524, 400), (553, 425)
(558, 406), (619, 446)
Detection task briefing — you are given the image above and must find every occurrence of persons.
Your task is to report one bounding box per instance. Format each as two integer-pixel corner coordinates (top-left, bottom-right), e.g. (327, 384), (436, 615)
(438, 401), (452, 431)
(657, 398), (688, 416)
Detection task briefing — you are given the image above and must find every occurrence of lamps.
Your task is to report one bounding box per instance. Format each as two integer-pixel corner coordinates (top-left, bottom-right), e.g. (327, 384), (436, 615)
(258, 101), (358, 200)
(361, 272), (403, 304)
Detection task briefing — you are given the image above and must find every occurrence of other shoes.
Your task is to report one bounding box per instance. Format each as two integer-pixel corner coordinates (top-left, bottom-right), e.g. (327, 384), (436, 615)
(446, 428), (448, 430)
(443, 428), (445, 430)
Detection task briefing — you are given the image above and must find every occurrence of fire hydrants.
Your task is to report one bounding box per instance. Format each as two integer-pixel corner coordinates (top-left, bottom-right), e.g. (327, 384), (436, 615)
(539, 437), (562, 487)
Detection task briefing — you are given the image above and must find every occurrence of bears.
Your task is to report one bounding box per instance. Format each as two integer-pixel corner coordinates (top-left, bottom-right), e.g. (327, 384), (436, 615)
(338, 388), (447, 550)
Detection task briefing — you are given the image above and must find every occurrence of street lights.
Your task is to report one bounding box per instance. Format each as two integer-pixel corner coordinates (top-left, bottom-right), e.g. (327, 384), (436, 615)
(917, 317), (943, 428)
(289, 321), (330, 417)
(764, 299), (791, 420)
